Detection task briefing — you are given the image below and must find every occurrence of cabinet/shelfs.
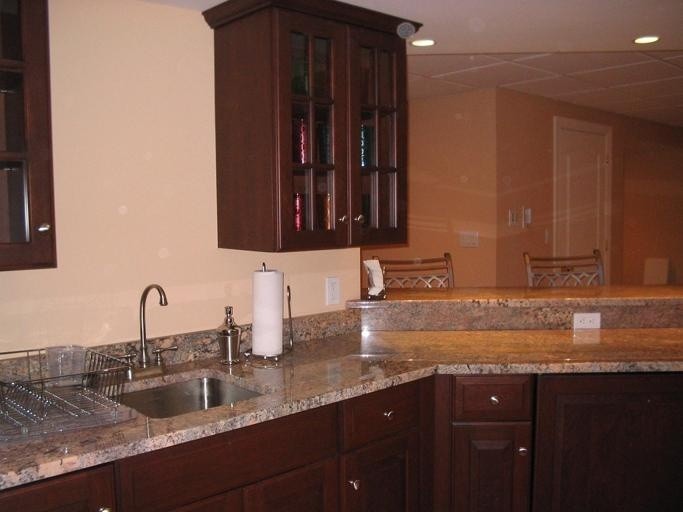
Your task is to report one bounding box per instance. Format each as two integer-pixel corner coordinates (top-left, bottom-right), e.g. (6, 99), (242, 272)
(437, 373), (532, 508)
(1, 380), (338, 510)
(201, 0), (424, 252)
(1, 2), (59, 273)
(537, 376), (683, 511)
(345, 381), (434, 508)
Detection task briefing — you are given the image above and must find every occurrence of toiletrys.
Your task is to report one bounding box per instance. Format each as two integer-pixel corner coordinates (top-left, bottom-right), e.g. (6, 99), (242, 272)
(217, 307), (242, 364)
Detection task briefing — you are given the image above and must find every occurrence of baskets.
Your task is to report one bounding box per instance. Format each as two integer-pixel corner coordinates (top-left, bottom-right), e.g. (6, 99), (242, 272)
(1, 346), (135, 442)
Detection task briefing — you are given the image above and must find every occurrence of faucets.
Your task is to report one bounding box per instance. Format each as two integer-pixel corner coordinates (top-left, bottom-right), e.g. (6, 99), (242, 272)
(139, 283), (168, 365)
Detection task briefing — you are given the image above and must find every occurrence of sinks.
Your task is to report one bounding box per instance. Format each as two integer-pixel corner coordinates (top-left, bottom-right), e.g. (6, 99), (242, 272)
(99, 369), (271, 419)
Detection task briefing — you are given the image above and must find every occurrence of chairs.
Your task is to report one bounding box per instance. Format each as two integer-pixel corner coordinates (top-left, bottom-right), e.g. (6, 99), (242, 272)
(517, 251), (610, 288)
(370, 253), (457, 293)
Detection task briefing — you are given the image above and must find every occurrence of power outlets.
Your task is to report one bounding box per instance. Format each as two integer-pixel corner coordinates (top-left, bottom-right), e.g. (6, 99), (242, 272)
(324, 276), (340, 307)
(573, 312), (603, 332)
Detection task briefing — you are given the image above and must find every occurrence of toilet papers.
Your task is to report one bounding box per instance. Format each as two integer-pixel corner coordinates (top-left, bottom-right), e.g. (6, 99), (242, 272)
(252, 271), (284, 357)
